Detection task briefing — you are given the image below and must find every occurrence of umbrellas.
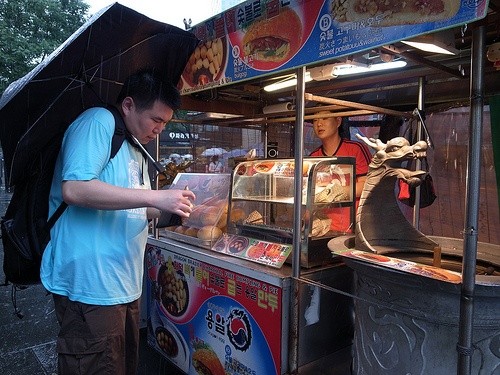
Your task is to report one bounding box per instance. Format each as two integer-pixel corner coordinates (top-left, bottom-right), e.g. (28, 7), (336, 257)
(169, 153), (193, 161)
(0, 2), (201, 193)
(201, 148), (247, 158)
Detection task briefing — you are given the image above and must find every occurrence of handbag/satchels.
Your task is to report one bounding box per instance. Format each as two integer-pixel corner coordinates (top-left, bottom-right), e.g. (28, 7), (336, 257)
(398, 172), (436, 208)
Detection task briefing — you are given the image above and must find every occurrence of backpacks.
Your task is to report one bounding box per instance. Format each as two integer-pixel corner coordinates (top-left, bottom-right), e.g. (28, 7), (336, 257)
(0, 105), (127, 319)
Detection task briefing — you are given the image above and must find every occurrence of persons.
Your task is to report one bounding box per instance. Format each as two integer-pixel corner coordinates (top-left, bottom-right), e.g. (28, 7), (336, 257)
(170, 155), (223, 174)
(303, 102), (375, 238)
(40, 70), (195, 375)
